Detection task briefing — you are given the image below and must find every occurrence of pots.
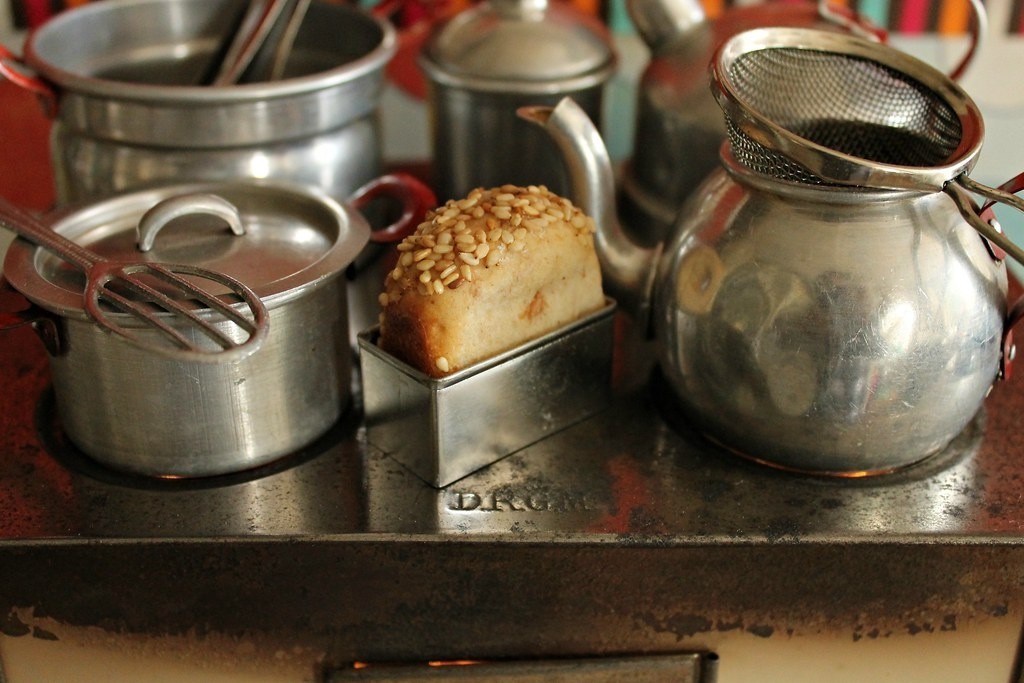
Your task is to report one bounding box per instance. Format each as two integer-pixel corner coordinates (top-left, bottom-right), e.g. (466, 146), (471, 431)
(5, 179), (370, 479)
(0, 0), (400, 275)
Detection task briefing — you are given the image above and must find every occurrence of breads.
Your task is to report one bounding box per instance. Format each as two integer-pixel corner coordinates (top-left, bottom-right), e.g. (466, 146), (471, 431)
(376, 183), (606, 378)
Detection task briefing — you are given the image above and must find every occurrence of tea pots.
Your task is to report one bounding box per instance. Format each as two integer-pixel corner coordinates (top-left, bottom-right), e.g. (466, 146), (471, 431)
(622, 0), (882, 231)
(514, 97), (1024, 475)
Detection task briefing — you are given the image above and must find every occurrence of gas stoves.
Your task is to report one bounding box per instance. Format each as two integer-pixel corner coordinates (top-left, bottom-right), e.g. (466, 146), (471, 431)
(0, 160), (1024, 683)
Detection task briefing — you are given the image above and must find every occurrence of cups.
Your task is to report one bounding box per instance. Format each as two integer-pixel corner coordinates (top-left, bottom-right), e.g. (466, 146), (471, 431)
(420, 0), (615, 209)
